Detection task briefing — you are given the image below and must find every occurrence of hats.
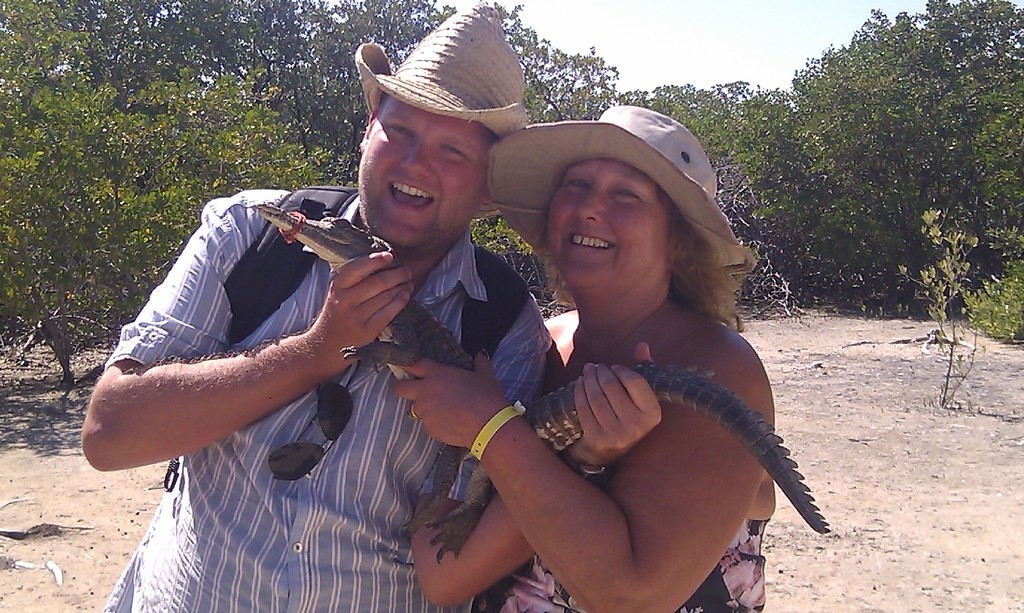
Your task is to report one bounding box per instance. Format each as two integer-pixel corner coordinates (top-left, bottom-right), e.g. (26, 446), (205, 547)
(354, 2), (530, 139)
(484, 105), (757, 275)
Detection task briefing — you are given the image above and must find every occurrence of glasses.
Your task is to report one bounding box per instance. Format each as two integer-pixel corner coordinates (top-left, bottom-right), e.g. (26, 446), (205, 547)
(268, 381), (353, 482)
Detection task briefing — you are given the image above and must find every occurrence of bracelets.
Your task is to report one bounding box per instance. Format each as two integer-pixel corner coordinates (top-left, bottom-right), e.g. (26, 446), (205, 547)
(566, 450), (607, 474)
(470, 401), (527, 460)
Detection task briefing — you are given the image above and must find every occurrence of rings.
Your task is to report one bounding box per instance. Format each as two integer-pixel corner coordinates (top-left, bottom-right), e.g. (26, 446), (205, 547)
(407, 404), (420, 419)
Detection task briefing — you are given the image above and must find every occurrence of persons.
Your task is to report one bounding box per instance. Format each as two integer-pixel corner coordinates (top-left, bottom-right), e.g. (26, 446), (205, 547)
(83, 15), (662, 613)
(393, 104), (775, 613)
